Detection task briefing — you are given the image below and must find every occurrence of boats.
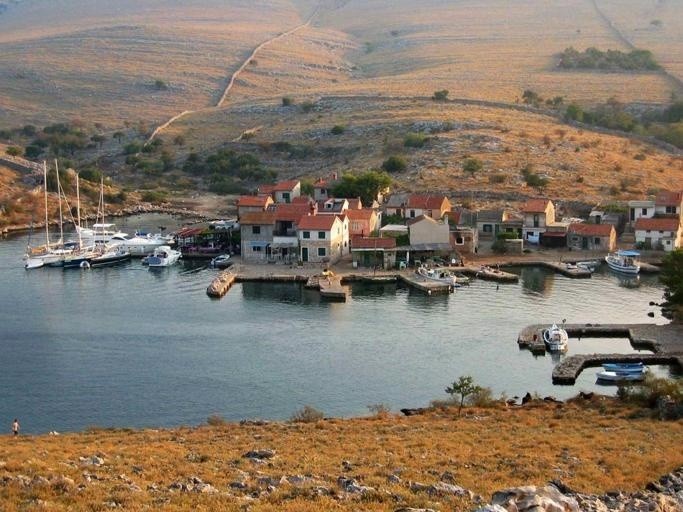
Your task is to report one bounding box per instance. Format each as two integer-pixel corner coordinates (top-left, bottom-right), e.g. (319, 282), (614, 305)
(544, 325), (568, 350)
(418, 265), (469, 286)
(596, 362), (646, 381)
(568, 250), (640, 273)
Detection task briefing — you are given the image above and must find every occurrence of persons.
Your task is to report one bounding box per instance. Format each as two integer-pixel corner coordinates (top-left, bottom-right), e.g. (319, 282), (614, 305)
(619, 257), (632, 267)
(11, 418), (19, 436)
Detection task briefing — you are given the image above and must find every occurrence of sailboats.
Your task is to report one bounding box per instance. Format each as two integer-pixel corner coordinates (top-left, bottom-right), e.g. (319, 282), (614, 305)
(23, 159), (232, 269)
